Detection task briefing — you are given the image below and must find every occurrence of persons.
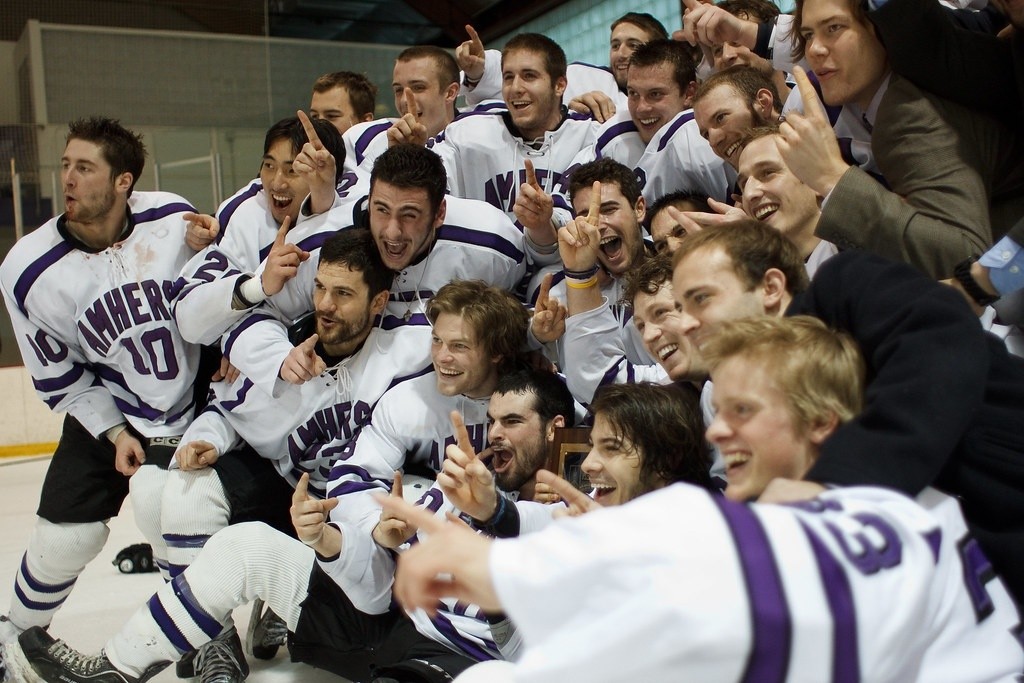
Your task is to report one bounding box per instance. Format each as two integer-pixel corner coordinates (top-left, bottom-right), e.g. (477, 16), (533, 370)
(0, 0), (1024, 683)
(672, 219), (1024, 615)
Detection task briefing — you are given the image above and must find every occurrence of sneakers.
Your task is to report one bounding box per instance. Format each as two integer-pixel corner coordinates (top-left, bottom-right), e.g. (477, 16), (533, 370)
(246, 596), (288, 661)
(110, 541), (161, 574)
(0, 614), (173, 683)
(193, 627), (250, 683)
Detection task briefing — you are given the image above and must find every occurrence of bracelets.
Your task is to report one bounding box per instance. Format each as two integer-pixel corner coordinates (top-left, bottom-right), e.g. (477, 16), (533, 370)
(566, 276), (599, 288)
(565, 273), (599, 283)
(563, 264), (597, 279)
(298, 531), (323, 544)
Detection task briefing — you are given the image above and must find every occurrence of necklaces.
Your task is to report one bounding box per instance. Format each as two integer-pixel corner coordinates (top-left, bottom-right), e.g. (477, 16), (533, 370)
(392, 227), (433, 322)
